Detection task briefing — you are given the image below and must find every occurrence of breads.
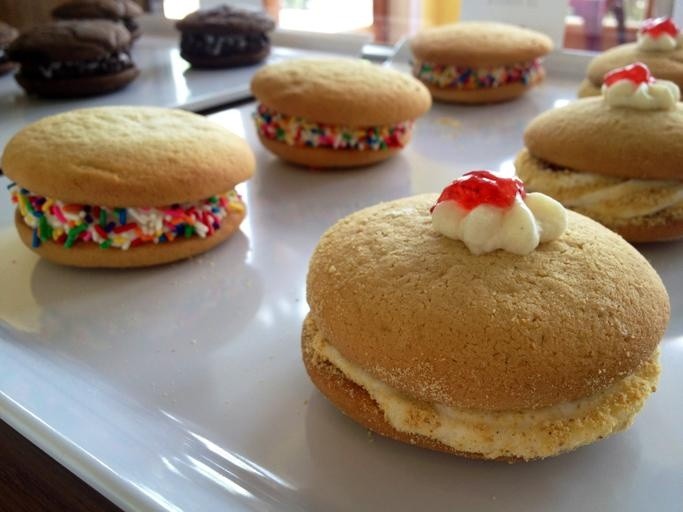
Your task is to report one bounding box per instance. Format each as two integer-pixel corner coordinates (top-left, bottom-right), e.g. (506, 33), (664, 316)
(513, 61), (683, 243)
(1, 104), (255, 268)
(410, 21), (554, 105)
(251, 57), (433, 171)
(300, 170), (672, 462)
(577, 15), (683, 100)
(175, 5), (275, 68)
(0, 0), (144, 99)
